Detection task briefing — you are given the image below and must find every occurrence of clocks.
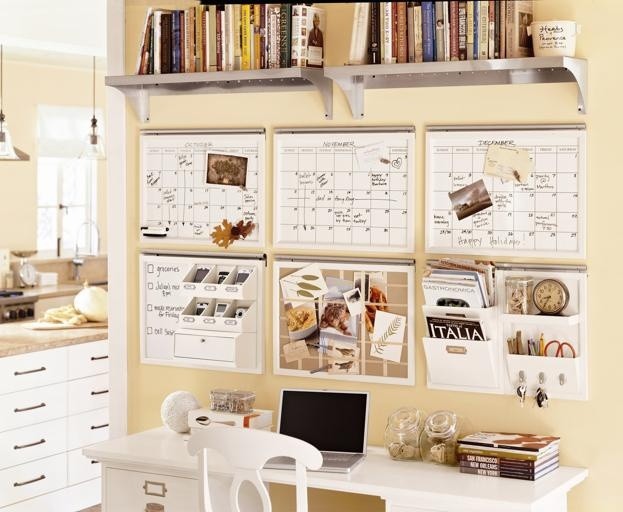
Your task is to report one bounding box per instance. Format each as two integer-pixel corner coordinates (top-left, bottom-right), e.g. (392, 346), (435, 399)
(532, 276), (568, 316)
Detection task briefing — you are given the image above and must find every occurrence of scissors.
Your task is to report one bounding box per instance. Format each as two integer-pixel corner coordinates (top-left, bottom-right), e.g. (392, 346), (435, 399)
(545, 340), (575, 358)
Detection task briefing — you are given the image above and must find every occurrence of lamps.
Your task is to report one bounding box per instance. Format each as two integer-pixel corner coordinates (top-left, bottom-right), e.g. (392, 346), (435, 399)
(0, 40), (30, 164)
(76, 55), (107, 161)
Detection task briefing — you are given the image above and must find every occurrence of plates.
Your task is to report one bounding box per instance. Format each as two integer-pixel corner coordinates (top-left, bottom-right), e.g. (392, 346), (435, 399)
(287, 303), (316, 339)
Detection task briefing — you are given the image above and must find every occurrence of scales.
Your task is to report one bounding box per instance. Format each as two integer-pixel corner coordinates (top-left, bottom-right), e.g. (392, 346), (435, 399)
(11, 250), (40, 289)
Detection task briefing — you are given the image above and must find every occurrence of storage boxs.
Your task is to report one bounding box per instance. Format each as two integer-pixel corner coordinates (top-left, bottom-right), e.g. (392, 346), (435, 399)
(187, 405), (273, 434)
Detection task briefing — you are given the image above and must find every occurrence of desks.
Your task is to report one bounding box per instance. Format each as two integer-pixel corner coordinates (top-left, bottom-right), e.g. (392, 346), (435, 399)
(82, 422), (589, 512)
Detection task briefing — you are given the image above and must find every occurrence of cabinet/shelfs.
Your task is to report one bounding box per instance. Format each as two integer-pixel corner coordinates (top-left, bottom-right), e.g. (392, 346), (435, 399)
(0, 339), (111, 512)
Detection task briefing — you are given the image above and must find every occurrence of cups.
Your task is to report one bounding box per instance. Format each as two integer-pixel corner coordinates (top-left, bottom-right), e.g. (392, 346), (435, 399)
(530, 20), (580, 58)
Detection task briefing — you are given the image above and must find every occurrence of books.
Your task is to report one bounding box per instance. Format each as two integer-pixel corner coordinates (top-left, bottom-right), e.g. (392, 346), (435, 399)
(457, 431), (562, 481)
(134, 3), (325, 76)
(420, 251), (497, 343)
(348, 0), (532, 64)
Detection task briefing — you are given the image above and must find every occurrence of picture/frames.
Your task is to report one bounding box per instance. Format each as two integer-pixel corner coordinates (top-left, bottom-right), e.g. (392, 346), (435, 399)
(271, 124), (417, 254)
(134, 252), (265, 379)
(269, 258), (417, 390)
(422, 122), (591, 262)
(134, 128), (265, 250)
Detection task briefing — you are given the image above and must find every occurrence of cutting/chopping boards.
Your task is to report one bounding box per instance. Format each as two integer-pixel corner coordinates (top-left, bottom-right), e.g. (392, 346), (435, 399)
(22, 322), (108, 331)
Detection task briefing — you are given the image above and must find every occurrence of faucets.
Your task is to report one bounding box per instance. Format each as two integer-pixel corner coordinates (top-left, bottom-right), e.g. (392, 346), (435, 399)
(69, 219), (101, 282)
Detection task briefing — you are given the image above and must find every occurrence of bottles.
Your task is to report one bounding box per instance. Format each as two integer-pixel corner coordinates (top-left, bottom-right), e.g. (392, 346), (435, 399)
(383, 406), (471, 466)
(505, 276), (535, 315)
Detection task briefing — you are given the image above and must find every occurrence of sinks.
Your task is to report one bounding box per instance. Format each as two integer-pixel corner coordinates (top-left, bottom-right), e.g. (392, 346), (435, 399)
(86, 279), (110, 289)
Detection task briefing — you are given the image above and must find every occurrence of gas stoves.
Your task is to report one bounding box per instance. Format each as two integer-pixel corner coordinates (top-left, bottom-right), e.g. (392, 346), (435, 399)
(0, 291), (38, 324)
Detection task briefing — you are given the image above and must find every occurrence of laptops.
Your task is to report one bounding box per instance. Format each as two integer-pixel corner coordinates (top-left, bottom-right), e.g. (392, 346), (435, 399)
(263, 388), (370, 472)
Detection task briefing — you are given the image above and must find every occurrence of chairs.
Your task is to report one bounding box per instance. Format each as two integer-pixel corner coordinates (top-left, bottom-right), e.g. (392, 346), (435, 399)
(184, 423), (325, 512)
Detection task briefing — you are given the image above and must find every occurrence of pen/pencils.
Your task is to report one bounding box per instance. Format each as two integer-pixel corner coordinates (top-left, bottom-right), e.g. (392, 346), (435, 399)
(528, 334), (545, 356)
